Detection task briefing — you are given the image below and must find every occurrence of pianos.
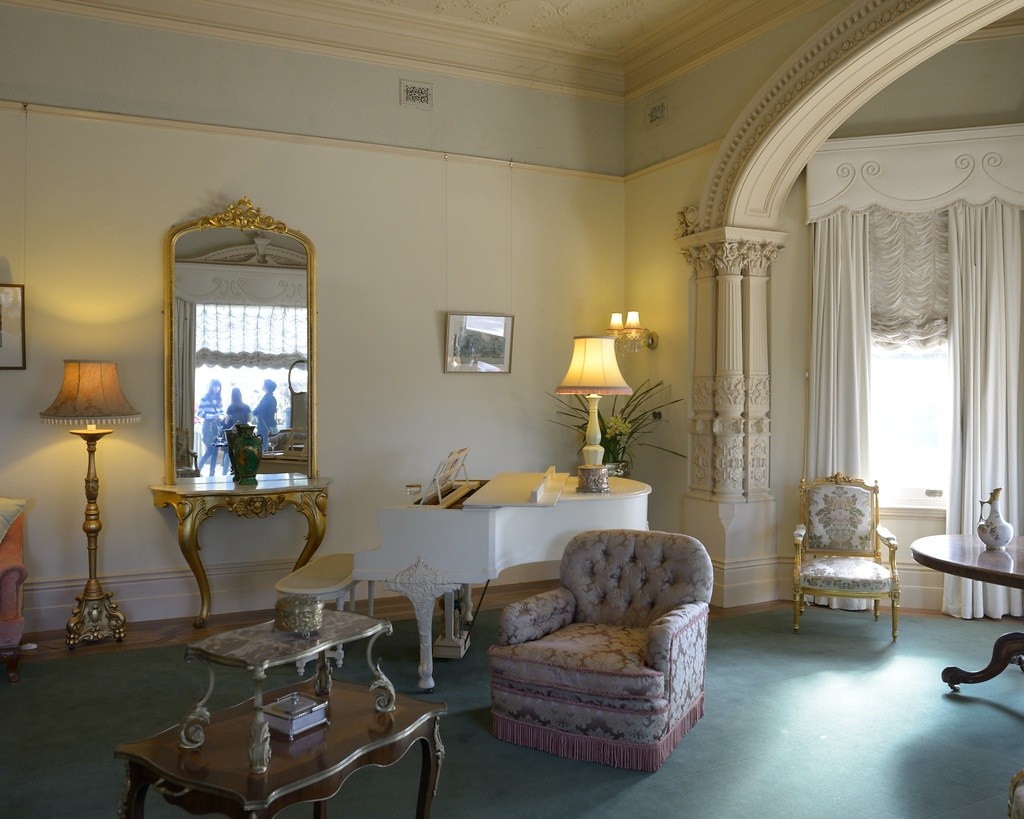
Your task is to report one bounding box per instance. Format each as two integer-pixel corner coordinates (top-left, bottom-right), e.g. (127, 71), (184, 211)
(352, 447), (652, 694)
(256, 429), (308, 475)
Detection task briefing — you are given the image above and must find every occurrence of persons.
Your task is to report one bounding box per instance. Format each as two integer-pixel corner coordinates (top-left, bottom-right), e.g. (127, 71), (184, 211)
(222, 388), (252, 475)
(253, 380), (277, 452)
(198, 379), (224, 477)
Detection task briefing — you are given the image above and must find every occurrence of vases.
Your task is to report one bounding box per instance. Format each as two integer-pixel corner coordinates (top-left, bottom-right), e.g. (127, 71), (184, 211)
(233, 426), (262, 484)
(225, 422), (253, 482)
(976, 487), (1013, 550)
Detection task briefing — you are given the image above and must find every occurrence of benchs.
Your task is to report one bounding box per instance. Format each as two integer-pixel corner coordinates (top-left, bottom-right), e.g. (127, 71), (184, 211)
(275, 553), (375, 676)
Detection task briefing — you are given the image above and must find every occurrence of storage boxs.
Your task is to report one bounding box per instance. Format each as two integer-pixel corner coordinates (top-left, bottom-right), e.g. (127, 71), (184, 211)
(261, 691), (331, 740)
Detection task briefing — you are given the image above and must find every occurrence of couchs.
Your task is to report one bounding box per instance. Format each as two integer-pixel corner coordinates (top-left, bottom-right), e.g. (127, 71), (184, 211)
(488, 528), (714, 771)
(0, 496), (29, 682)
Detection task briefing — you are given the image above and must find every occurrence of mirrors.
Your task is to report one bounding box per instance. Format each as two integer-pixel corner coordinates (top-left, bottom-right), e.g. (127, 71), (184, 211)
(162, 197), (318, 484)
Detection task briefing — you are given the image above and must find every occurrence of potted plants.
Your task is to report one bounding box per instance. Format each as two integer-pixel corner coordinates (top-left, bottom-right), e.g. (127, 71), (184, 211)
(544, 378), (688, 479)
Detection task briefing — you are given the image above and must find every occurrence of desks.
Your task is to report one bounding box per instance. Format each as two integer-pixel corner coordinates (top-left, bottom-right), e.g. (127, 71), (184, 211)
(148, 478), (333, 628)
(114, 671), (448, 819)
(180, 607), (397, 775)
(908, 534), (1024, 691)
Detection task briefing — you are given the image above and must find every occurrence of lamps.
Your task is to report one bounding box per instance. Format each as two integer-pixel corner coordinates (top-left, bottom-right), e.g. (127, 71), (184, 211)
(40, 359), (144, 651)
(556, 335), (633, 494)
(605, 311), (658, 353)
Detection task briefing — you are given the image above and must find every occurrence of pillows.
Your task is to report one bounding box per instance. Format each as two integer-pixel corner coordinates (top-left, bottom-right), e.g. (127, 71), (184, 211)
(0, 498), (27, 545)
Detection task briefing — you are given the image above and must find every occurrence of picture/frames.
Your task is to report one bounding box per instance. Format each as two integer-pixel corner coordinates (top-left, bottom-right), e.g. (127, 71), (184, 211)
(444, 312), (514, 374)
(0, 283), (26, 370)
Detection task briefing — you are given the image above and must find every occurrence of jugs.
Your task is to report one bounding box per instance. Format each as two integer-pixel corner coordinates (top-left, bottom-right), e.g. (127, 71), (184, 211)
(975, 488), (1014, 551)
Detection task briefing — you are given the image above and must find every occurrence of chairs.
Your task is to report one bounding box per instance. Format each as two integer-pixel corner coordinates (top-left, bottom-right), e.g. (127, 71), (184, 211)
(175, 427), (200, 478)
(792, 472), (902, 643)
